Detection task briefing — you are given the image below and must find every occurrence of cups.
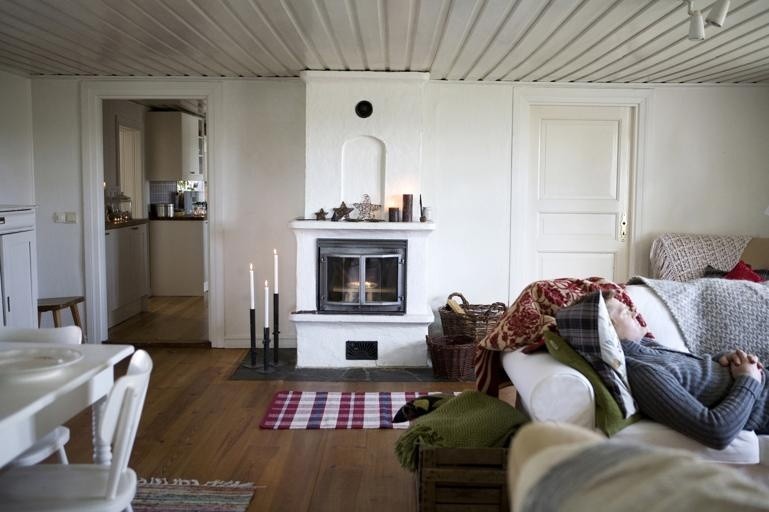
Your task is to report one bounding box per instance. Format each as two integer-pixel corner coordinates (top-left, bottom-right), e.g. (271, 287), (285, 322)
(156, 203), (167, 218)
(389, 208), (398, 222)
(167, 204), (175, 217)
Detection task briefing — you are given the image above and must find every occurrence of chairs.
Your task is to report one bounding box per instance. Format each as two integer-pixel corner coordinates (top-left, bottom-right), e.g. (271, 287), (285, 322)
(1, 348), (154, 512)
(0, 325), (84, 466)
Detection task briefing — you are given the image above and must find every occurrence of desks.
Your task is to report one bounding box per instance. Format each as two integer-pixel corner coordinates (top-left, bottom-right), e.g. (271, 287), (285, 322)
(149, 214), (208, 297)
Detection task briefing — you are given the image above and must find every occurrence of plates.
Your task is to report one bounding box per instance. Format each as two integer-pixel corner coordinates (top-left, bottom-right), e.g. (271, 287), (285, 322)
(0, 346), (83, 373)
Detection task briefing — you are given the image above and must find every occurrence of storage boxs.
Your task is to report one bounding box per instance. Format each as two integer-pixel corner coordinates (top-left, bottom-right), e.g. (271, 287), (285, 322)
(416, 446), (507, 512)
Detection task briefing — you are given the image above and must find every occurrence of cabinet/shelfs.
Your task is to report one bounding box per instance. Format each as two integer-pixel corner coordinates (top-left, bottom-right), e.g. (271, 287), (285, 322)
(146, 111), (207, 181)
(0, 209), (38, 329)
(106, 224), (148, 328)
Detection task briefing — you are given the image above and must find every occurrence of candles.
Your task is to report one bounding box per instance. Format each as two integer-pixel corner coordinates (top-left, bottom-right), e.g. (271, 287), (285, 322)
(249, 250), (279, 329)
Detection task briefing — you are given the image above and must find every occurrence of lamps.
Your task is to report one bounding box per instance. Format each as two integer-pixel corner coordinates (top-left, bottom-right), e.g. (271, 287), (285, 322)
(686, 1), (705, 41)
(706, 0), (730, 27)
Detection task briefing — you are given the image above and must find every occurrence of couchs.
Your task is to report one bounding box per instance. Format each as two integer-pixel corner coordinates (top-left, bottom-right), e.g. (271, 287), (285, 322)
(502, 278), (768, 466)
(507, 420), (768, 512)
(649, 233), (769, 282)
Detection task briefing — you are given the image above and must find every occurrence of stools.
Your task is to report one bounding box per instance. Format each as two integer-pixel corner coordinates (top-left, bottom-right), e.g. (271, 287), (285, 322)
(38, 296), (86, 344)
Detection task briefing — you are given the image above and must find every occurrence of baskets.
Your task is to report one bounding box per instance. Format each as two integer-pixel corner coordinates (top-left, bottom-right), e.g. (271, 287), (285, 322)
(427, 334), (476, 379)
(438, 293), (507, 344)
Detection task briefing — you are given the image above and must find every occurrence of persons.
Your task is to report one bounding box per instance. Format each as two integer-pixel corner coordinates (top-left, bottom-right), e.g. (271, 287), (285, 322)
(569, 288), (769, 450)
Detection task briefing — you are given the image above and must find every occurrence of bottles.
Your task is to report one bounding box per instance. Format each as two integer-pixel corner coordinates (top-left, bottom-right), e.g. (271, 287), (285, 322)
(175, 192), (179, 208)
(179, 193), (184, 209)
(402, 194), (413, 222)
(424, 207), (432, 220)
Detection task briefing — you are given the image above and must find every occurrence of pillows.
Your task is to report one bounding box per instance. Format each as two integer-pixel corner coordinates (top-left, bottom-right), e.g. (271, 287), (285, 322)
(542, 331), (640, 439)
(555, 288), (641, 420)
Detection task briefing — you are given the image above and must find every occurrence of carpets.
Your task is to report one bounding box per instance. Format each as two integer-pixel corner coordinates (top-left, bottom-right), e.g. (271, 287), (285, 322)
(229, 348), (444, 382)
(121, 475), (257, 512)
(260, 390), (465, 430)
